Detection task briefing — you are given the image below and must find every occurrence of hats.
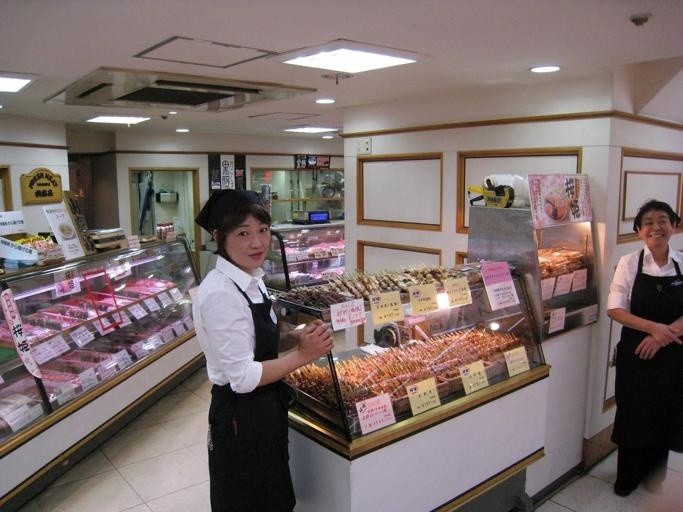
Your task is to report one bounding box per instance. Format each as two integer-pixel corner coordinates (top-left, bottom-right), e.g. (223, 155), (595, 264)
(194, 189), (264, 233)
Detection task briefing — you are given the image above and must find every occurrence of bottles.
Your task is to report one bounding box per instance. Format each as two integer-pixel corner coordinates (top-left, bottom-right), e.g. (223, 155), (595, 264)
(156, 222), (174, 240)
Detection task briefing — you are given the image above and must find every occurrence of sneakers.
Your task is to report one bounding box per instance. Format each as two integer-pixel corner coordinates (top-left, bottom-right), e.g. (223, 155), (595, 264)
(645, 479), (664, 495)
(613, 478), (638, 496)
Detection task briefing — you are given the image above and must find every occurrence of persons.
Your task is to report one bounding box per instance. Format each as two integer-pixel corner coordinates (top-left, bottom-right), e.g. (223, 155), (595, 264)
(192, 188), (334, 512)
(605, 199), (683, 498)
